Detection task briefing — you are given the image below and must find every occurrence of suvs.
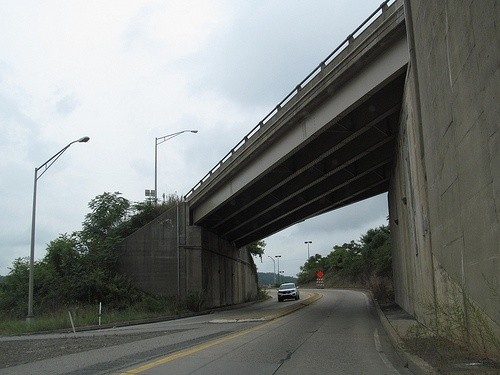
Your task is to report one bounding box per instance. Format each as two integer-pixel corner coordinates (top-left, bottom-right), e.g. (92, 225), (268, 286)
(277, 283), (299, 302)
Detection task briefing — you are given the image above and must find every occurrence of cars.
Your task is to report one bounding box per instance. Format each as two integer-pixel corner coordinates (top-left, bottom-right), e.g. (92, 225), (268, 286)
(267, 286), (271, 289)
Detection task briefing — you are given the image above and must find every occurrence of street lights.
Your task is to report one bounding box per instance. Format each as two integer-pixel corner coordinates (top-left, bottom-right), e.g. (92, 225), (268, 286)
(26, 137), (90, 318)
(275, 256), (281, 283)
(154, 130), (198, 208)
(305, 241), (312, 261)
(268, 256), (275, 284)
